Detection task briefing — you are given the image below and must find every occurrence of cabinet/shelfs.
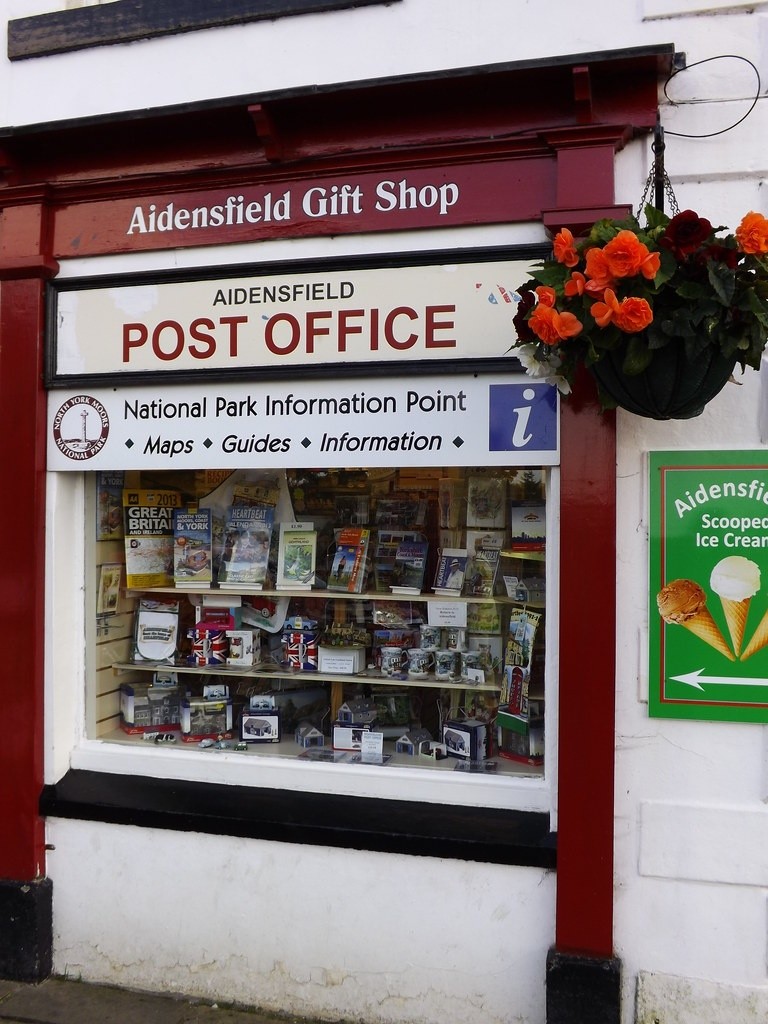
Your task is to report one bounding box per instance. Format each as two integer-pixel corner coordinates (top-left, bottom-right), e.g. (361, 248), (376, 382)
(86, 576), (555, 780)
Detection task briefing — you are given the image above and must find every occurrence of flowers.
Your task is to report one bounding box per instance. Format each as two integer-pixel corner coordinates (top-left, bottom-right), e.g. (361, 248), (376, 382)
(504, 210), (767, 409)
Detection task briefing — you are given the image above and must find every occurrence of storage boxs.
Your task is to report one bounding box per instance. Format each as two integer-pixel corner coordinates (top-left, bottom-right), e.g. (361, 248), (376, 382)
(96, 471), (547, 775)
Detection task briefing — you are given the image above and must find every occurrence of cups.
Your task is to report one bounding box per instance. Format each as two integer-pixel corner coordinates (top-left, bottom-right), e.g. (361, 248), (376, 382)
(407, 648), (435, 680)
(461, 651), (478, 677)
(380, 648), (408, 677)
(435, 650), (461, 681)
(420, 624), (441, 651)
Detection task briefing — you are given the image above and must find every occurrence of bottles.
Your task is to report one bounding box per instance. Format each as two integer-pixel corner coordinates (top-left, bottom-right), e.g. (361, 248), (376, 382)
(447, 628), (467, 652)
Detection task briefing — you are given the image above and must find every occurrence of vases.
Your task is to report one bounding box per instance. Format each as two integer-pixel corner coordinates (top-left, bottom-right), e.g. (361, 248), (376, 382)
(578, 311), (751, 422)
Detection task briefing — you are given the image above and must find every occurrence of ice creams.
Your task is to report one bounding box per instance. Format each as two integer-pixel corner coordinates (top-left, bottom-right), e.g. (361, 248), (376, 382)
(656, 556), (768, 662)
